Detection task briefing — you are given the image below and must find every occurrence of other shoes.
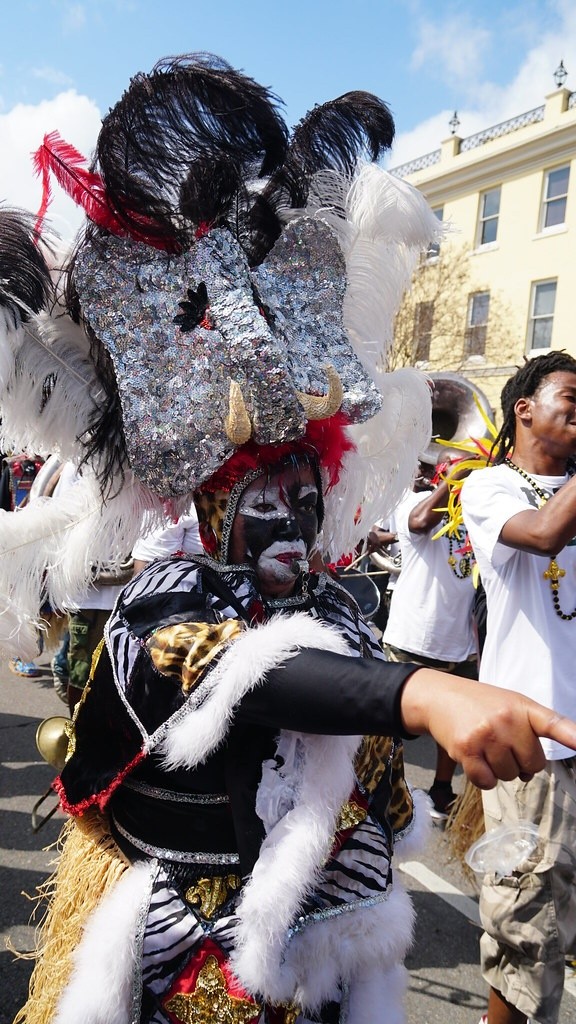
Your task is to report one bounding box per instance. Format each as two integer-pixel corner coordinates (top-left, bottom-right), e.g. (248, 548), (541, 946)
(8, 657), (39, 676)
(429, 793), (471, 828)
(52, 655), (74, 705)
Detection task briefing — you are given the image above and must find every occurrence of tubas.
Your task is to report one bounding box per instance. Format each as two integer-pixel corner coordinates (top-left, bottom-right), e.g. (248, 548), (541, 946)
(417, 371), (494, 466)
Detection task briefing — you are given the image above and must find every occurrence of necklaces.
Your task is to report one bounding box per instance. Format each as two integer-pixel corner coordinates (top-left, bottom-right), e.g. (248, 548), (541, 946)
(505, 458), (576, 619)
(448, 516), (470, 579)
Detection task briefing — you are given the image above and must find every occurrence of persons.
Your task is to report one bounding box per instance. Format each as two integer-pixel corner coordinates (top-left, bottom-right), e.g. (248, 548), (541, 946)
(460, 351), (576, 1023)
(0, 448), (207, 728)
(382, 458), (483, 819)
(43, 444), (576, 1023)
(368, 462), (437, 614)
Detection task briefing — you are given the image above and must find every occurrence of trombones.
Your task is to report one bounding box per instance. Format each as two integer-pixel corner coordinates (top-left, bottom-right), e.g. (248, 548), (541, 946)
(30, 715), (77, 835)
